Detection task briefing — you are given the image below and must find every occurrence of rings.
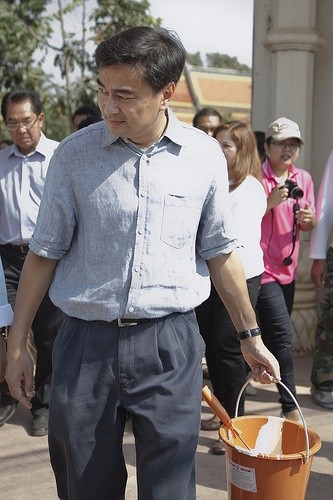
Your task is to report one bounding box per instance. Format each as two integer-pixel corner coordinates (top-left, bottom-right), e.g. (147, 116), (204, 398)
(305, 217), (310, 223)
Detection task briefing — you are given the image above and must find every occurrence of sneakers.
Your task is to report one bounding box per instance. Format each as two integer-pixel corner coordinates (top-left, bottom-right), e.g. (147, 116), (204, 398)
(311, 386), (333, 408)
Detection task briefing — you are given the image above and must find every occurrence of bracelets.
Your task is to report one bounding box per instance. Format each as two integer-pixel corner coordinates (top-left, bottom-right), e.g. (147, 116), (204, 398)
(235, 328), (262, 341)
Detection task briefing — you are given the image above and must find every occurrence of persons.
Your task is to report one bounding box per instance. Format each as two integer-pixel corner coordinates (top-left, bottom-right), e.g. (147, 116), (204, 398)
(201, 120), (267, 455)
(5, 25), (281, 500)
(242, 117), (316, 433)
(68, 103), (225, 140)
(311, 149), (333, 406)
(0, 90), (65, 437)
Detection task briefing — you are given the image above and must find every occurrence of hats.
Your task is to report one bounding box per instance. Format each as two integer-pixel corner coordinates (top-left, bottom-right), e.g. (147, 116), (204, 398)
(265, 116), (303, 143)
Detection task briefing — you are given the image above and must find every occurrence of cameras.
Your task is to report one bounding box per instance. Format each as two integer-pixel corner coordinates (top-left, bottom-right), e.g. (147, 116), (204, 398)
(278, 180), (304, 199)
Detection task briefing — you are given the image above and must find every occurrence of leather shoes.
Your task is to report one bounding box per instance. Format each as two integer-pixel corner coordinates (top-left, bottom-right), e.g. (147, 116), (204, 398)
(0, 403), (17, 426)
(212, 440), (226, 455)
(201, 416), (221, 430)
(30, 415), (48, 435)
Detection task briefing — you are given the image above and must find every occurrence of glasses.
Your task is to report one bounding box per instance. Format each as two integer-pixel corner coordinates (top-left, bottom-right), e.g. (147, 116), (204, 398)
(6, 116), (37, 131)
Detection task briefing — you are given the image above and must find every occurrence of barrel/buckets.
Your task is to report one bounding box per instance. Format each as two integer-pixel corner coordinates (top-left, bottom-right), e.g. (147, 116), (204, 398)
(218, 376), (322, 500)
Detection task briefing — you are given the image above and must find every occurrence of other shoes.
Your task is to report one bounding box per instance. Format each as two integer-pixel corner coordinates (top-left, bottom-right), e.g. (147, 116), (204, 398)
(246, 383), (258, 395)
(281, 411), (307, 425)
(203, 373), (211, 379)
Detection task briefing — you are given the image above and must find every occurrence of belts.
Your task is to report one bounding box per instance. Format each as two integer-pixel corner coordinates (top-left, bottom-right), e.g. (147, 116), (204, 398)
(10, 244), (29, 254)
(110, 317), (151, 326)
